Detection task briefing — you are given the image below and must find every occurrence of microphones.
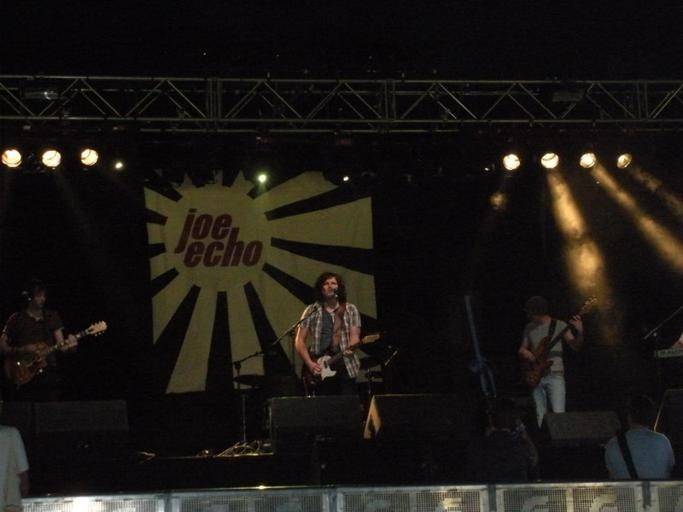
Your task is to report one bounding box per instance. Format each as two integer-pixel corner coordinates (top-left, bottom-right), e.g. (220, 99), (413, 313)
(21, 290), (32, 301)
(333, 284), (339, 299)
(385, 351), (398, 366)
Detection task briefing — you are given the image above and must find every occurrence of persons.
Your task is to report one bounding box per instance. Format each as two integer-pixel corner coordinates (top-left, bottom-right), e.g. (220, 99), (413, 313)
(1, 279), (78, 392)
(515, 296), (587, 430)
(290, 271), (363, 395)
(0, 402), (33, 498)
(469, 396), (537, 484)
(602, 392), (676, 482)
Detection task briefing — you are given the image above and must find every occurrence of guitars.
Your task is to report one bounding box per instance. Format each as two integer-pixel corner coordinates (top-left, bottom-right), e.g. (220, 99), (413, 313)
(301, 334), (380, 383)
(0, 321), (107, 389)
(523, 295), (598, 388)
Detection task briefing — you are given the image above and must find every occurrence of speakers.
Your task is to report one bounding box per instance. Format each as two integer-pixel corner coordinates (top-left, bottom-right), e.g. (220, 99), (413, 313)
(33, 400), (130, 455)
(268, 396), (362, 455)
(0, 400), (31, 457)
(541, 412), (622, 449)
(362, 395), (464, 440)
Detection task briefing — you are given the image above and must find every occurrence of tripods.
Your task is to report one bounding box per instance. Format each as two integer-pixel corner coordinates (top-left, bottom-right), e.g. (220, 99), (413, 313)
(216, 343), (272, 455)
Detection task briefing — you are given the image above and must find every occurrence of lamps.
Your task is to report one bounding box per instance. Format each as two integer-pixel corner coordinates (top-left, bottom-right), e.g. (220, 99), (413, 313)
(265, 393), (364, 461)
(537, 410), (627, 463)
(358, 388), (476, 444)
